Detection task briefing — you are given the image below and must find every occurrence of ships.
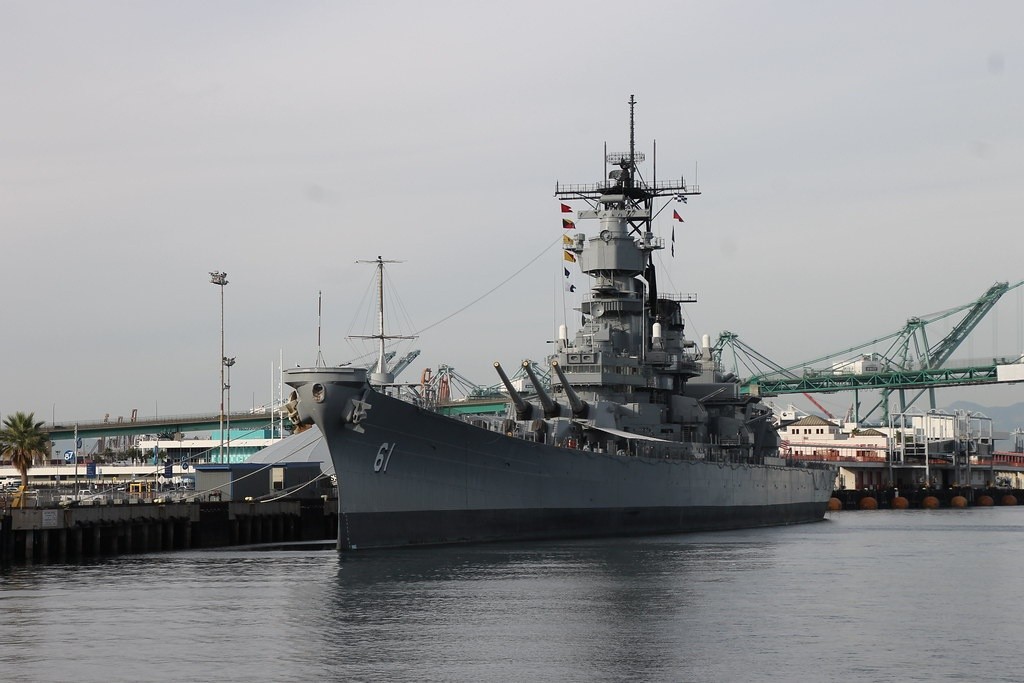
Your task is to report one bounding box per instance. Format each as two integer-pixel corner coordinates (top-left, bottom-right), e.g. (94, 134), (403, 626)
(281, 92), (843, 549)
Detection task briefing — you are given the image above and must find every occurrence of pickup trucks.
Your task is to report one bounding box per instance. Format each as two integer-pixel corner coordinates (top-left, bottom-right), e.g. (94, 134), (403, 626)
(60, 490), (104, 505)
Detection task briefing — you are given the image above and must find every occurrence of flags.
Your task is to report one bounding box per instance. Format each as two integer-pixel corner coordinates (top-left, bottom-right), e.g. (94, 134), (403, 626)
(563, 234), (576, 248)
(564, 251), (576, 263)
(563, 219), (575, 228)
(565, 268), (570, 278)
